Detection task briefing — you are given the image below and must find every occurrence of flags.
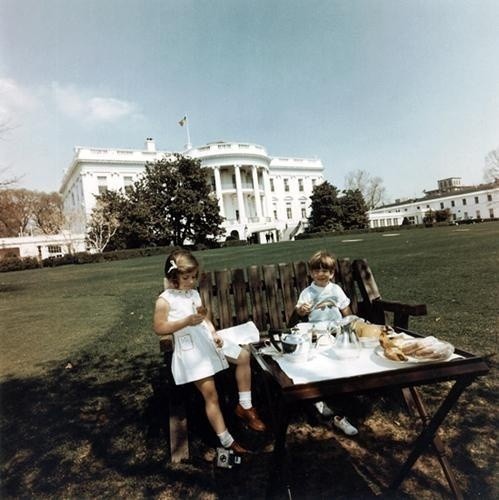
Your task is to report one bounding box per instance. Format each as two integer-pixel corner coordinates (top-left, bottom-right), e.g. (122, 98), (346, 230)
(176, 115), (188, 129)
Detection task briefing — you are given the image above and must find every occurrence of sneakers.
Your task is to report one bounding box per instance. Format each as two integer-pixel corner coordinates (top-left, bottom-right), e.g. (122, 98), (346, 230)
(334, 415), (359, 436)
(314, 402), (333, 416)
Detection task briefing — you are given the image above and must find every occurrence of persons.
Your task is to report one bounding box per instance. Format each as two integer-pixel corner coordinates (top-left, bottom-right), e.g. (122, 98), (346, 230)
(153, 250), (265, 454)
(296, 250), (353, 416)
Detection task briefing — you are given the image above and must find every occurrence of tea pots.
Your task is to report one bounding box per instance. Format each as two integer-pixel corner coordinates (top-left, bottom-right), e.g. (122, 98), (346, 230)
(323, 316), (363, 358)
(268, 327), (323, 361)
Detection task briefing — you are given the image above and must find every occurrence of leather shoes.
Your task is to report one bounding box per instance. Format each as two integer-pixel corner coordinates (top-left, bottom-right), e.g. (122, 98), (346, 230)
(235, 404), (264, 431)
(224, 441), (247, 458)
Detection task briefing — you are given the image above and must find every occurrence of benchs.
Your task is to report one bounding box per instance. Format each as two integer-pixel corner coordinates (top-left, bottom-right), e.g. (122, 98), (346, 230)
(158, 257), (428, 464)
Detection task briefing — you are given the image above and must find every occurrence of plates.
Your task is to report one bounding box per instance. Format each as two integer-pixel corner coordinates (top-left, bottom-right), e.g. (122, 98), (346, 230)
(375, 343), (454, 364)
